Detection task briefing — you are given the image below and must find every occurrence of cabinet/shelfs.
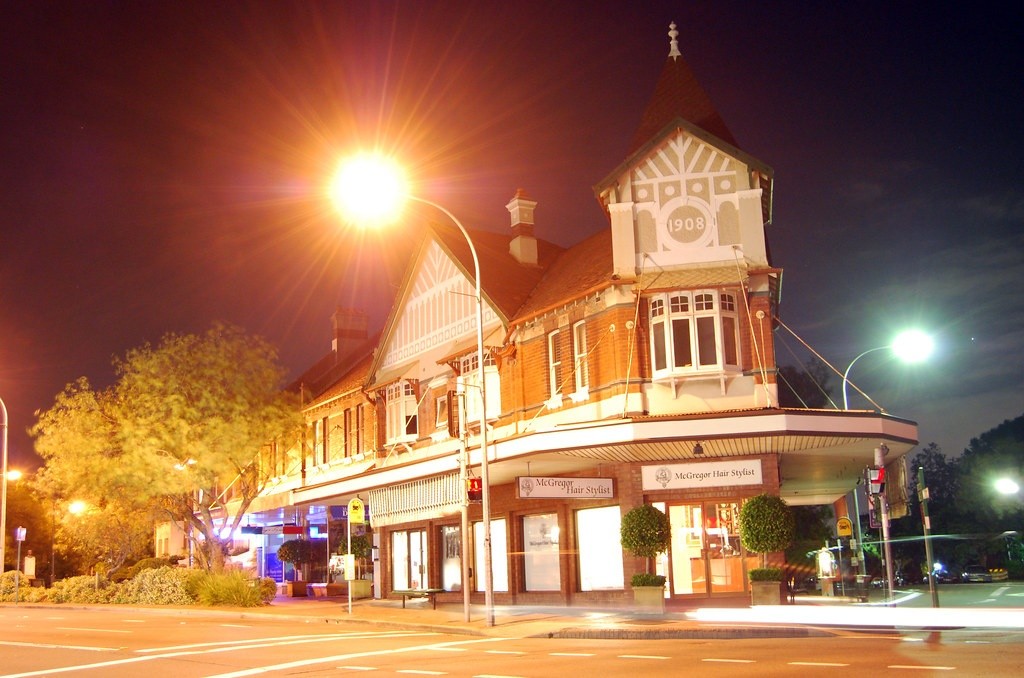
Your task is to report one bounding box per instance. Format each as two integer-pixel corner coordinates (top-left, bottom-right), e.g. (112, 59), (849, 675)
(690, 554), (761, 592)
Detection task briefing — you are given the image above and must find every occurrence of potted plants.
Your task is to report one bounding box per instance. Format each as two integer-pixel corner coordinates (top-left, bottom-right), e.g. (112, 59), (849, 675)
(737, 491), (795, 603)
(619, 504), (671, 614)
(276, 538), (316, 598)
(339, 533), (373, 598)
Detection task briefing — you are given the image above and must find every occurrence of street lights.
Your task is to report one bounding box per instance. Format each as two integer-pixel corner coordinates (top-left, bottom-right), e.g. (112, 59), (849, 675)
(13, 525), (27, 604)
(345, 166), (496, 629)
(841, 330), (929, 415)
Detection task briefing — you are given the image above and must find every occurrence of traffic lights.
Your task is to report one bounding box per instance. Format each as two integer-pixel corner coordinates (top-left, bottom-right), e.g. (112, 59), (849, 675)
(468, 477), (484, 504)
(866, 465), (887, 498)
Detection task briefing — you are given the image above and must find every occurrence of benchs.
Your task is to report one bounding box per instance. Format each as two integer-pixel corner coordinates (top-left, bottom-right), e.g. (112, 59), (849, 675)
(391, 588), (445, 609)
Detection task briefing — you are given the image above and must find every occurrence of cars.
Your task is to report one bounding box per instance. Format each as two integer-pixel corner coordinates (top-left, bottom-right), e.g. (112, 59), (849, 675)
(924, 570), (958, 584)
(961, 564), (992, 583)
(870, 576), (889, 587)
